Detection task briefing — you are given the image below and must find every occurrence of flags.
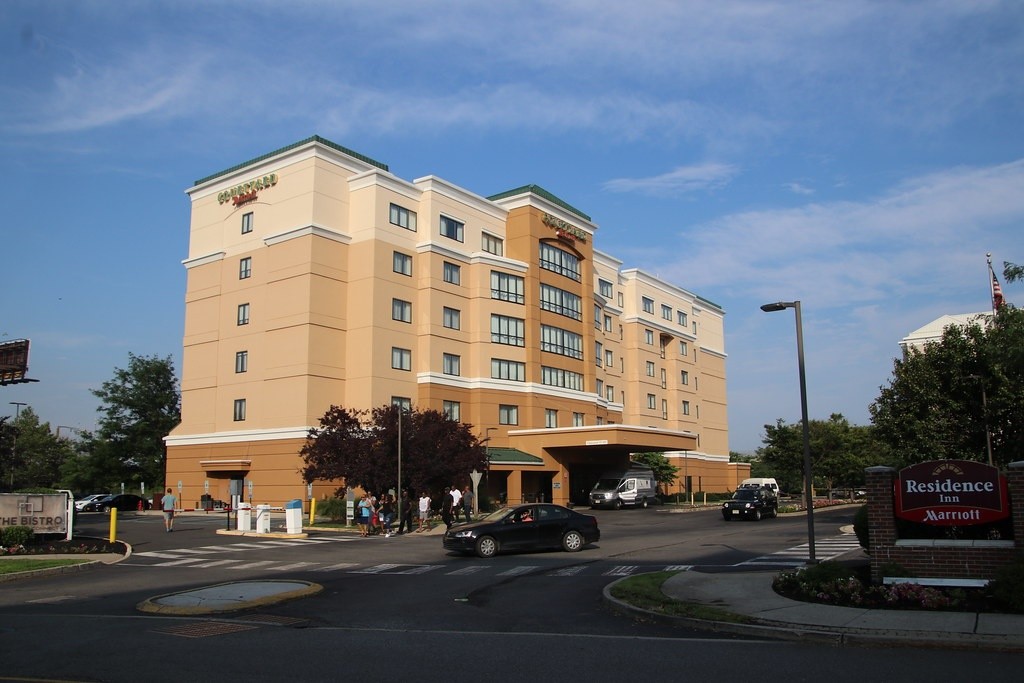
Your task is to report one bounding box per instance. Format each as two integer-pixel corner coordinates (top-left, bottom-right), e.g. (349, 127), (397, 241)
(989, 265), (1005, 311)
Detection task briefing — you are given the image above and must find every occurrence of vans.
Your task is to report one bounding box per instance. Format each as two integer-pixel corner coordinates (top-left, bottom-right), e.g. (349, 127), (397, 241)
(736, 478), (780, 498)
(590, 469), (655, 510)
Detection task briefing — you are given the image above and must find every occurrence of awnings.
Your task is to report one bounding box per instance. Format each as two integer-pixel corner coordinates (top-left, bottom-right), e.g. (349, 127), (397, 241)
(480, 447), (545, 466)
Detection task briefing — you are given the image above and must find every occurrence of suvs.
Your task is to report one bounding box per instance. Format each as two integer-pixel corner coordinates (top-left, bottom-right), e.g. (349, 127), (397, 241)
(721, 486), (778, 522)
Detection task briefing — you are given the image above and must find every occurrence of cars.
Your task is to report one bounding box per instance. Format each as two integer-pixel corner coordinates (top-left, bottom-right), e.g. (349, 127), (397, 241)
(74, 494), (112, 512)
(86, 494), (149, 513)
(442, 503), (601, 558)
(148, 499), (154, 509)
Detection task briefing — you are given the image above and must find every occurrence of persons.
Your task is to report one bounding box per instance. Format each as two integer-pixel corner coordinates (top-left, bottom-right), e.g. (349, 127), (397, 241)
(367, 492), (377, 533)
(442, 487), (453, 529)
(416, 492), (431, 532)
(378, 494), (385, 535)
(462, 486), (473, 523)
(376, 495), (396, 538)
(396, 491), (412, 536)
(449, 485), (462, 522)
(161, 488), (178, 533)
(356, 494), (371, 537)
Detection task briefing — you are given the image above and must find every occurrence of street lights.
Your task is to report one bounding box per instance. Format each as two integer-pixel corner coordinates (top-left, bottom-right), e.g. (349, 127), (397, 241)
(486, 427), (498, 483)
(8, 401), (28, 485)
(397, 396), (411, 520)
(760, 301), (821, 566)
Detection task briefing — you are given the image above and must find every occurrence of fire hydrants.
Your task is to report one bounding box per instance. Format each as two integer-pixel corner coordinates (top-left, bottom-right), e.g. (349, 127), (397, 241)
(137, 500), (143, 511)
